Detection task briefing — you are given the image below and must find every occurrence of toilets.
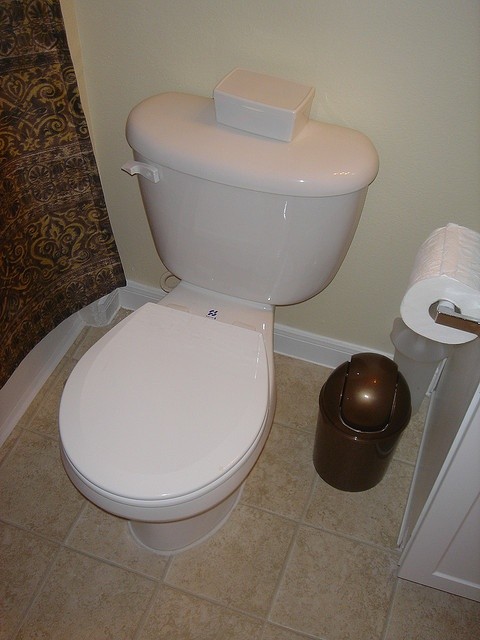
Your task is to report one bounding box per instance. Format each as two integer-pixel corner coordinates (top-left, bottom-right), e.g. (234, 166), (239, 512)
(58, 93), (380, 556)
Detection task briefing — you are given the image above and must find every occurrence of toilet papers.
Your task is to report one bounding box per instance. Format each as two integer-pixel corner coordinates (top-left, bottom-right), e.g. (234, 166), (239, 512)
(400, 223), (480, 346)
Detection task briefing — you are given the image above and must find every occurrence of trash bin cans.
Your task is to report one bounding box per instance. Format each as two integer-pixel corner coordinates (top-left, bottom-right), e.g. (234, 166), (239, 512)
(312, 352), (412, 493)
